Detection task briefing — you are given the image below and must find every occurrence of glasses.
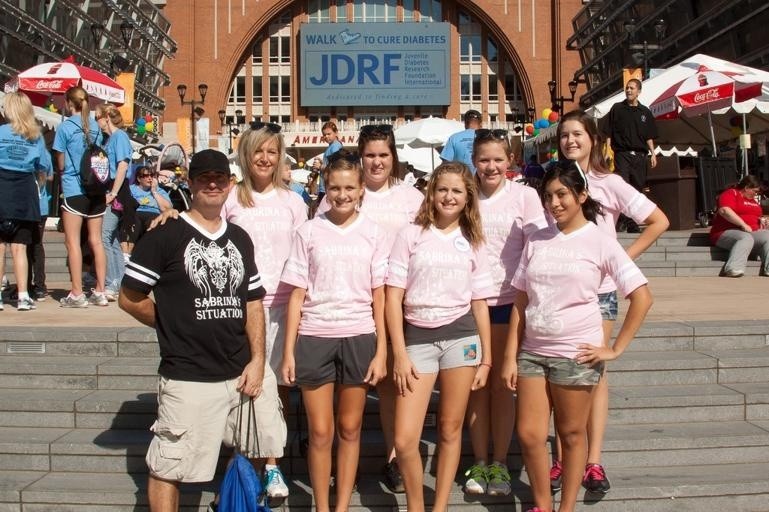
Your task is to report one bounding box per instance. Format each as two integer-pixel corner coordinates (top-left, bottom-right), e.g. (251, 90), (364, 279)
(249, 121), (281, 133)
(545, 159), (587, 190)
(360, 125), (392, 135)
(474, 129), (511, 146)
(331, 152), (357, 162)
(143, 174), (154, 178)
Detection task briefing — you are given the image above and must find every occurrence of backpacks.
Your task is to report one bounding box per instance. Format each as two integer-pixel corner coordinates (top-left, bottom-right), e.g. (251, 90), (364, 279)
(64, 120), (110, 196)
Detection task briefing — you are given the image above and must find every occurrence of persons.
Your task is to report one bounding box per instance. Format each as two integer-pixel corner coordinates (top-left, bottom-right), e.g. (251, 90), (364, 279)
(382, 163), (494, 512)
(1, 78), (657, 313)
(210, 122), (308, 498)
(312, 120), (432, 495)
(499, 162), (654, 512)
(707, 174), (769, 279)
(463, 131), (552, 496)
(539, 112), (675, 498)
(112, 150), (288, 511)
(276, 150), (389, 512)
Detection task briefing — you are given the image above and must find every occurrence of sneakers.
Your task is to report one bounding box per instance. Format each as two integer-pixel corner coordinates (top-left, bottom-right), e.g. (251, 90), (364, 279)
(0, 271), (119, 310)
(262, 457), (609, 499)
(616, 221), (640, 232)
(719, 269), (743, 277)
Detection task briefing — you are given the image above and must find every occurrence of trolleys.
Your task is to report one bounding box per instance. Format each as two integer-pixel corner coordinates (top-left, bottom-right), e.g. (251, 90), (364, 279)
(699, 175), (750, 227)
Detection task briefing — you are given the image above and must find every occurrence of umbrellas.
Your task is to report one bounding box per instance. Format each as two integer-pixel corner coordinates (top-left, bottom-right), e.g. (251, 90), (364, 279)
(5, 53), (124, 216)
(647, 63), (765, 160)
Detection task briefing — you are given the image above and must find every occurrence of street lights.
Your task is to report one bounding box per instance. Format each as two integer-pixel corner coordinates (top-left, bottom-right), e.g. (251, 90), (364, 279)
(511, 106), (535, 141)
(218, 110), (242, 156)
(547, 80), (578, 121)
(90, 20), (137, 81)
(624, 19), (666, 80)
(177, 83), (208, 161)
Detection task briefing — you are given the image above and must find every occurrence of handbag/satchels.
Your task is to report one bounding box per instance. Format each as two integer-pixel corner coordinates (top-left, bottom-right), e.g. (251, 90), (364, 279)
(219, 455), (270, 511)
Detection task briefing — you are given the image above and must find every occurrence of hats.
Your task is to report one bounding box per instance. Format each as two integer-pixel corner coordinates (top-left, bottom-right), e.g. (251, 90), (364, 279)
(189, 149), (230, 178)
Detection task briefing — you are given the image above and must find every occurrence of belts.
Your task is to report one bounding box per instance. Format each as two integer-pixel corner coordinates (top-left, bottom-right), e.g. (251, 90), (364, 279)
(624, 151), (644, 155)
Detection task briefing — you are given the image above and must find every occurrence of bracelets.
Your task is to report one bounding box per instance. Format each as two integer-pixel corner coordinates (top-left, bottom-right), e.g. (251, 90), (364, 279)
(479, 362), (493, 368)
